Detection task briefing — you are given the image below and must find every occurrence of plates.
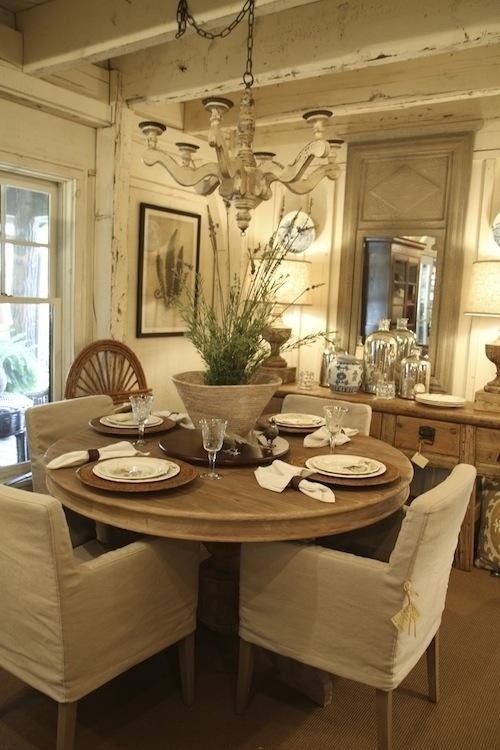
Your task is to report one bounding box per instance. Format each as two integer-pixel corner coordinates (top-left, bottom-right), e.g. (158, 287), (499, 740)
(88, 413), (176, 437)
(73, 456), (198, 494)
(255, 411), (326, 435)
(292, 454), (400, 488)
(278, 210), (316, 254)
(414, 393), (468, 409)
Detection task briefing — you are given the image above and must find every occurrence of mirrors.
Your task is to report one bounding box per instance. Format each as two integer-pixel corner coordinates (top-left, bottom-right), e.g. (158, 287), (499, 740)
(355, 235), (437, 361)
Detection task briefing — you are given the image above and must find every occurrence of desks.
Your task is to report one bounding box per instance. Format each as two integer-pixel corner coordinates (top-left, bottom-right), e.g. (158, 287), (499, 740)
(260, 383), (499, 575)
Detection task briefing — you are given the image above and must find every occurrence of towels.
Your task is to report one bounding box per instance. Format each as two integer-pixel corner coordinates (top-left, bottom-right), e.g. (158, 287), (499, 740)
(254, 455), (336, 504)
(154, 410), (196, 432)
(46, 439), (153, 473)
(301, 424), (361, 449)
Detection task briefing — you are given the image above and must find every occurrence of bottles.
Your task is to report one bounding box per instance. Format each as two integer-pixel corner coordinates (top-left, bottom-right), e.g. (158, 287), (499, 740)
(355, 335), (364, 360)
(364, 317), (432, 400)
(326, 356), (364, 395)
(320, 352), (331, 387)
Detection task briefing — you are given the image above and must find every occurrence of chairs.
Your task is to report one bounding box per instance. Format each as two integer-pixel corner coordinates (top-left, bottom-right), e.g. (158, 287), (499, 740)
(232, 461), (480, 749)
(0, 483), (203, 750)
(24, 392), (117, 547)
(278, 390), (375, 438)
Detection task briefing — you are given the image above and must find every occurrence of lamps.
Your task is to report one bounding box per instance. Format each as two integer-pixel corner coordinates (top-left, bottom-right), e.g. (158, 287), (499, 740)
(238, 254), (316, 386)
(138, 0), (343, 236)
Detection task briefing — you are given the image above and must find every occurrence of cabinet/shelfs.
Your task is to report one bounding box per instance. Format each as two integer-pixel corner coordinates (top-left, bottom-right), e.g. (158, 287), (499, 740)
(363, 235), (426, 338)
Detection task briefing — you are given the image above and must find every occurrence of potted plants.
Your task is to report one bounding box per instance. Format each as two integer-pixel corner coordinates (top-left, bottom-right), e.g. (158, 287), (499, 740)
(156, 193), (340, 446)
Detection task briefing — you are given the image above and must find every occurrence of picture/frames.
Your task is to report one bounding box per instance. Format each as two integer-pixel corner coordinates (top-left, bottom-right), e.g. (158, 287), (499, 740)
(135, 201), (202, 338)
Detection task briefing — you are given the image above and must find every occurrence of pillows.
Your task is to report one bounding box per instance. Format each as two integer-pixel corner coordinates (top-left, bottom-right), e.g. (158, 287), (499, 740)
(473, 475), (500, 576)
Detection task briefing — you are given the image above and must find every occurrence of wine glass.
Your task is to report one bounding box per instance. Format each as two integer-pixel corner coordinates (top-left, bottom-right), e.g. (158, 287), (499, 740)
(261, 327), (292, 367)
(197, 417), (229, 482)
(323, 405), (348, 453)
(482, 344), (500, 393)
(128, 392), (157, 448)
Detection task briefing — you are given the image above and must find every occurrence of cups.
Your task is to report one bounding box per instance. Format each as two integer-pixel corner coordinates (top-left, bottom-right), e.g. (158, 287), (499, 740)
(297, 370), (315, 391)
